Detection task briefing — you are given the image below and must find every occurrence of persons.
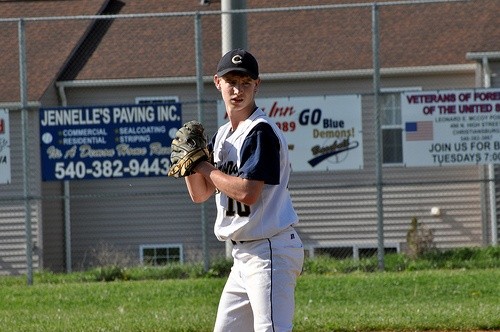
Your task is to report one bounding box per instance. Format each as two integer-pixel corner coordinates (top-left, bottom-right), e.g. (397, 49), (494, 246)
(171, 48), (304, 332)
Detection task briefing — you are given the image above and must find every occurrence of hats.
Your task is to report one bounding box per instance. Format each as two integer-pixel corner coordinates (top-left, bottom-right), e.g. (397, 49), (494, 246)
(217, 48), (258, 80)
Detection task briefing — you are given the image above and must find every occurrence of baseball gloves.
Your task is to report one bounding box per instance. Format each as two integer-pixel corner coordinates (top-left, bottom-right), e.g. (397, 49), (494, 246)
(165, 121), (210, 180)
